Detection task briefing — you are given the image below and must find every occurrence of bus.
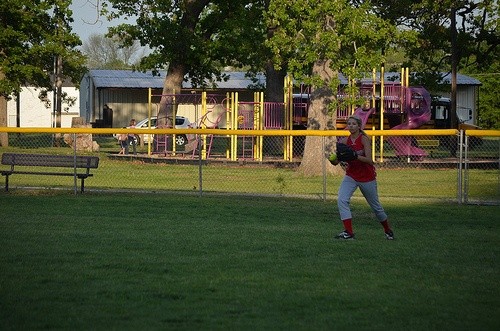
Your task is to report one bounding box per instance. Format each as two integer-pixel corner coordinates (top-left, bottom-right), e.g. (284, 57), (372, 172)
(288, 92), (482, 155)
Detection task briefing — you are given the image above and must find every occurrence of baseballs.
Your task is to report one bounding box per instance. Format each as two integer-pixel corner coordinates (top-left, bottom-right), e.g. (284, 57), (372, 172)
(328, 153), (337, 162)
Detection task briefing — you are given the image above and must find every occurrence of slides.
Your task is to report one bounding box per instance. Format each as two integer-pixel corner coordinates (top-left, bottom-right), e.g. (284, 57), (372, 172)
(385, 88), (432, 157)
(337, 107), (374, 144)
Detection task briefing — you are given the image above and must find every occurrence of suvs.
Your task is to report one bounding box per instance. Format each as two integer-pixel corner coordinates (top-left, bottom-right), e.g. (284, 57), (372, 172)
(112, 116), (196, 146)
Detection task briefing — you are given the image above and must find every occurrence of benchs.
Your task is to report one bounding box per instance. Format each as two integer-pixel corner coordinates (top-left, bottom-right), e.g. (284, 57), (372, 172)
(0, 153), (99, 194)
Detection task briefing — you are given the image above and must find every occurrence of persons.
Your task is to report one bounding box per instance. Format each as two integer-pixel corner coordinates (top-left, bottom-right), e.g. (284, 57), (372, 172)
(324, 115), (395, 241)
(117, 118), (138, 155)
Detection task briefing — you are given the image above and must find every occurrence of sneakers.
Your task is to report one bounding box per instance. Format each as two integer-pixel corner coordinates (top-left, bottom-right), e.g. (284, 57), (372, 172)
(385, 232), (394, 240)
(335, 232), (353, 240)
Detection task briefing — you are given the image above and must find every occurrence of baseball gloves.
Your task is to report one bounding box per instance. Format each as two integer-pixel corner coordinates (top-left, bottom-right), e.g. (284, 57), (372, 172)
(336, 142), (358, 162)
